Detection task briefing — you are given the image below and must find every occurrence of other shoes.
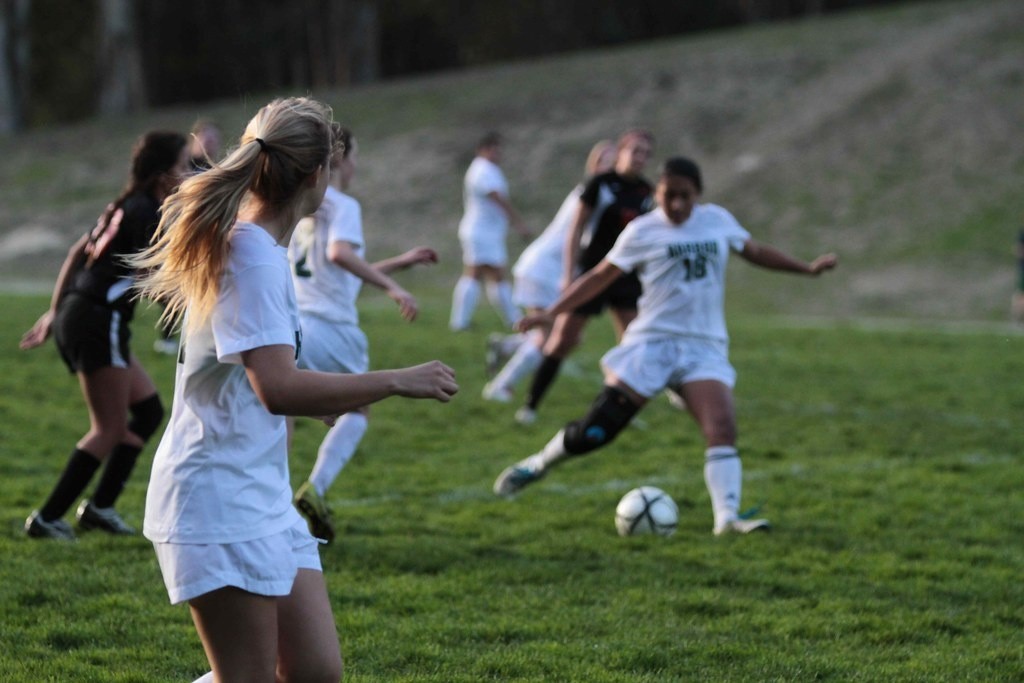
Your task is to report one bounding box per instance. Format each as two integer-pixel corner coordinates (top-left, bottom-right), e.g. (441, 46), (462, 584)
(485, 332), (502, 365)
(513, 403), (534, 424)
(493, 455), (542, 495)
(482, 382), (514, 402)
(713, 517), (772, 536)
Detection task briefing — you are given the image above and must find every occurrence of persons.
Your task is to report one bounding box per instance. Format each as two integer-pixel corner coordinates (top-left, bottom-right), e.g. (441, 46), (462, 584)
(116, 93), (460, 683)
(491, 158), (839, 537)
(22, 118), (438, 547)
(449, 130), (682, 422)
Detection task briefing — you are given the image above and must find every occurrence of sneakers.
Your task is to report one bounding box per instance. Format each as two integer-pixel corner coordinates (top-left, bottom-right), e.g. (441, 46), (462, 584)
(24, 511), (72, 538)
(295, 483), (332, 551)
(76, 502), (138, 537)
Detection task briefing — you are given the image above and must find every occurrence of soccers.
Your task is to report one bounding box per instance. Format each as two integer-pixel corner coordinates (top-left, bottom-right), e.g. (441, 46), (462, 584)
(614, 486), (681, 541)
